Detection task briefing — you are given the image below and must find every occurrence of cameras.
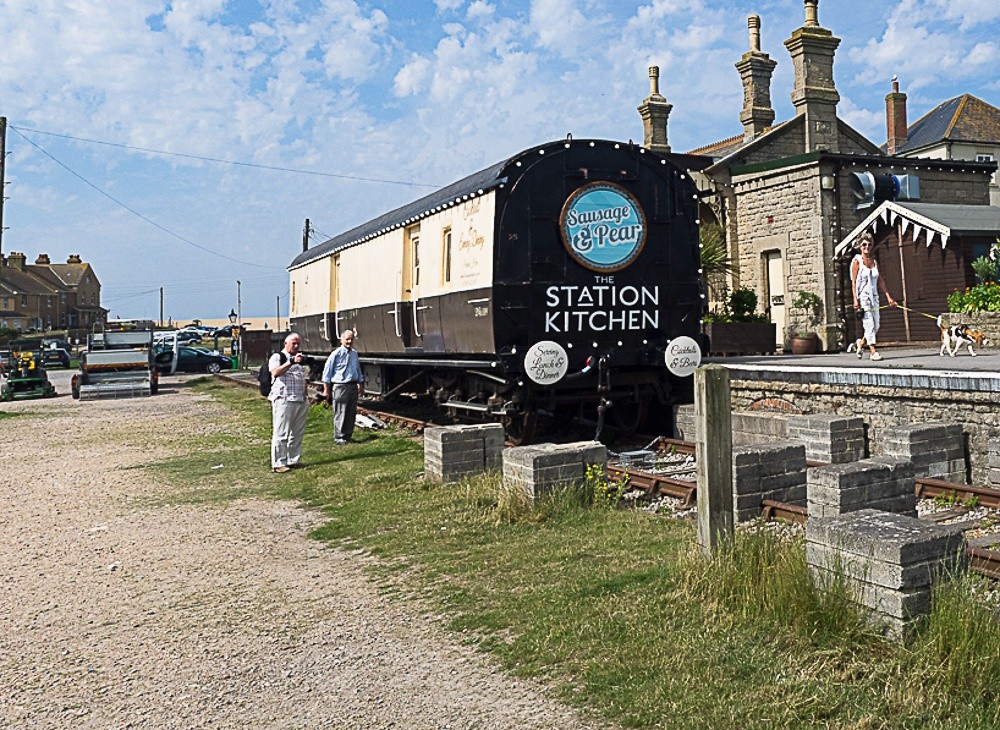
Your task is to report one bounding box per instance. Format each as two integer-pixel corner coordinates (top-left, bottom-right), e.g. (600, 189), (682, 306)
(856, 308), (864, 318)
(298, 356), (314, 365)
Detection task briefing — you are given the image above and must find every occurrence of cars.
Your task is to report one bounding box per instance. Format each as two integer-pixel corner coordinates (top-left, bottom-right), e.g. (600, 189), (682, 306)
(211, 325), (246, 337)
(0, 350), (12, 373)
(155, 346), (233, 376)
(42, 338), (78, 369)
(153, 326), (210, 346)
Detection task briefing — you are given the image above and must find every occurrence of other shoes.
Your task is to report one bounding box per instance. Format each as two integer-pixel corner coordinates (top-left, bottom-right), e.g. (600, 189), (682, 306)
(869, 352), (884, 360)
(271, 466), (292, 473)
(288, 463), (305, 468)
(334, 436), (362, 446)
(856, 338), (863, 359)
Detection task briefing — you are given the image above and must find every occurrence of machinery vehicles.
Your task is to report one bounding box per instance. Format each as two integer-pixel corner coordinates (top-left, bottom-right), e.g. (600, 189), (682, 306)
(71, 322), (158, 401)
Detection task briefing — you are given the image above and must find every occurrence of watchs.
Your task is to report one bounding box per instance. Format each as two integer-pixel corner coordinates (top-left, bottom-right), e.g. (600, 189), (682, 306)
(290, 356), (295, 364)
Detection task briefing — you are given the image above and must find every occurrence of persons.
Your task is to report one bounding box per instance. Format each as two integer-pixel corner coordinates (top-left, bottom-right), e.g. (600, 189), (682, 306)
(258, 332), (311, 473)
(321, 329), (365, 446)
(850, 233), (895, 361)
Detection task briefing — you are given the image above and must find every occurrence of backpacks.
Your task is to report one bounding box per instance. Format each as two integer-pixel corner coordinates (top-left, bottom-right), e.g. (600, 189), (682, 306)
(257, 352), (287, 396)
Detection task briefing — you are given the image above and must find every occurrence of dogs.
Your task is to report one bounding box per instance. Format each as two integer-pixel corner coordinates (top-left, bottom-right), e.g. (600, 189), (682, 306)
(937, 314), (989, 357)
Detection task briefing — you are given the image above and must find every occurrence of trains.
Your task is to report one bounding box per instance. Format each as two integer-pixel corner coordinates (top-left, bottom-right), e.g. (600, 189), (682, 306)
(287, 133), (711, 452)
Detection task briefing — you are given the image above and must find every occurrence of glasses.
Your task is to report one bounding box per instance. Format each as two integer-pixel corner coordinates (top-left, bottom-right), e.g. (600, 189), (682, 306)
(861, 243), (872, 246)
